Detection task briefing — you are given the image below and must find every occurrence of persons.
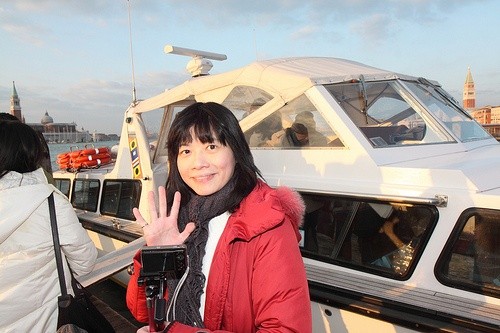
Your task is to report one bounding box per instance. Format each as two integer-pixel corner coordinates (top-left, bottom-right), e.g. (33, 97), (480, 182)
(241, 98), (282, 148)
(361, 202), (415, 255)
(269, 111), (330, 147)
(0, 123), (99, 333)
(126, 102), (312, 333)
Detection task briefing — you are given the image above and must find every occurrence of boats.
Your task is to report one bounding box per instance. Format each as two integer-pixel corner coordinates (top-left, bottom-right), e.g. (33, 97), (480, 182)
(48, 44), (500, 332)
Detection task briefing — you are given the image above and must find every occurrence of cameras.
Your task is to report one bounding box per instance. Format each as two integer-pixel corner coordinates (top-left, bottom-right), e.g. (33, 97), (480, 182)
(140, 244), (187, 278)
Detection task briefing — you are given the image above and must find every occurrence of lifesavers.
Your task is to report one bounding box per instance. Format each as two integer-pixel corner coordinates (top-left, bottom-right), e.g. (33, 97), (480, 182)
(56, 147), (111, 169)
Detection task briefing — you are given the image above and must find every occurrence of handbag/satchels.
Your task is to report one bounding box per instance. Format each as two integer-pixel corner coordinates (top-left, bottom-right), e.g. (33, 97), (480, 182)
(55, 290), (116, 333)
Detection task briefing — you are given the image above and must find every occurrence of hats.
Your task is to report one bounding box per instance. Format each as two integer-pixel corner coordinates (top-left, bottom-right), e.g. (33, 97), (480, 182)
(292, 117), (317, 136)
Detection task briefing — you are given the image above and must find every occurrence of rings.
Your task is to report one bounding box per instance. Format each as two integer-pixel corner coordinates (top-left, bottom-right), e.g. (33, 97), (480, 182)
(141, 223), (148, 229)
